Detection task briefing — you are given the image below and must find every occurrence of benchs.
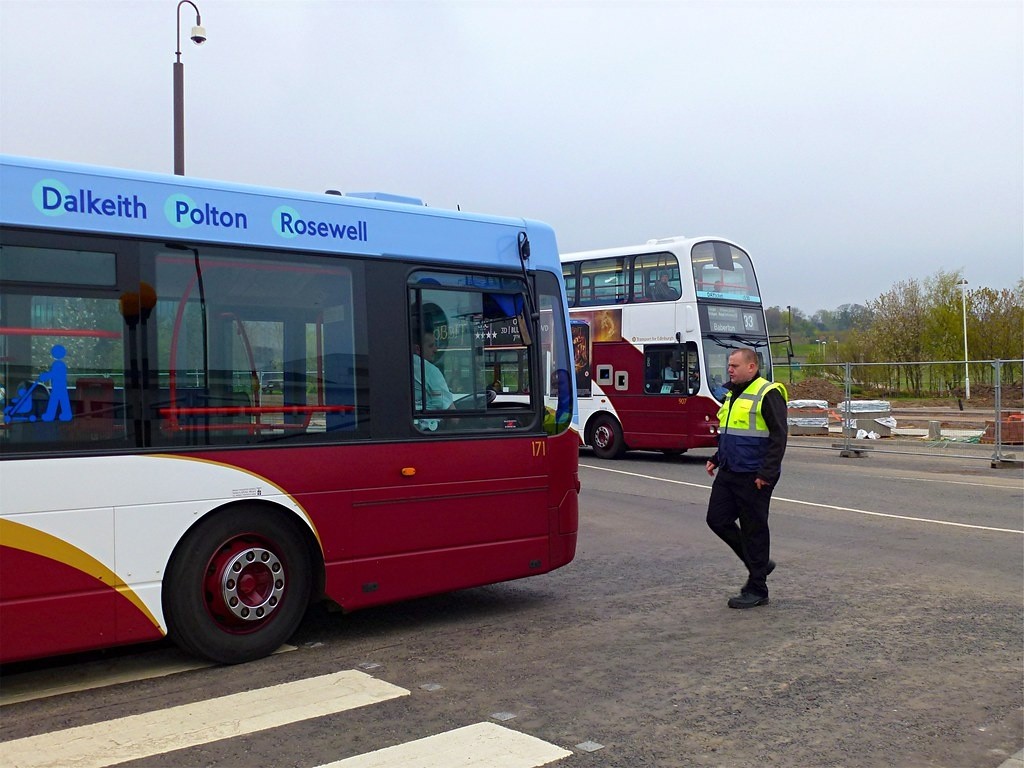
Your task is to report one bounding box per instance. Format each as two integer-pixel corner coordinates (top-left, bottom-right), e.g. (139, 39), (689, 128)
(567, 285), (678, 307)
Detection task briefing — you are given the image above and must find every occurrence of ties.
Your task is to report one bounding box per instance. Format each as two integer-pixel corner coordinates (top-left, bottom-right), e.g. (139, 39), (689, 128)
(670, 369), (677, 378)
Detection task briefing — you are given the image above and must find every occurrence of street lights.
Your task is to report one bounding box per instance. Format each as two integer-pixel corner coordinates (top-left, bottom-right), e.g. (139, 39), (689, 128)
(957, 278), (970, 399)
(786, 306), (790, 345)
(173, 0), (206, 173)
(815, 340), (821, 360)
(822, 342), (826, 360)
(834, 341), (839, 374)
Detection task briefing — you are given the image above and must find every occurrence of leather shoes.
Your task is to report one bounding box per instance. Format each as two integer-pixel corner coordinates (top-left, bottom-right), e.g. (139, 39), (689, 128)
(740, 560), (775, 591)
(727, 588), (769, 609)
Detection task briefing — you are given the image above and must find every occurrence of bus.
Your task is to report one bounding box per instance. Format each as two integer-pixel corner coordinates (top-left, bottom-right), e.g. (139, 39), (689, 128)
(442, 235), (795, 459)
(0, 153), (581, 671)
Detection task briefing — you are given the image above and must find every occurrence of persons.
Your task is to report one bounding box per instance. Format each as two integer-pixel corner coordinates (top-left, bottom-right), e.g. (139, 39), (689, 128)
(705, 348), (788, 609)
(414, 325), (460, 426)
(654, 271), (677, 300)
(661, 356), (684, 381)
